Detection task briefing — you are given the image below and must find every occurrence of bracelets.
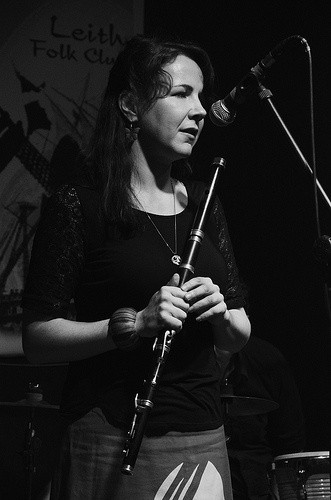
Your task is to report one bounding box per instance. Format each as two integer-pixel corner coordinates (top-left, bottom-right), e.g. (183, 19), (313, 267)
(108, 306), (147, 354)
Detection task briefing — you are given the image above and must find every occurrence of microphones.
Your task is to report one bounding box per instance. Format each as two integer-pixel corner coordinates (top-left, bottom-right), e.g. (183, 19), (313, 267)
(208, 35), (292, 129)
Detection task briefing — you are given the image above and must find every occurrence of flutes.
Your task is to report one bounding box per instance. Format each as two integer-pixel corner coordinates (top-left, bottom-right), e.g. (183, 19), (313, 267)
(120, 156), (227, 475)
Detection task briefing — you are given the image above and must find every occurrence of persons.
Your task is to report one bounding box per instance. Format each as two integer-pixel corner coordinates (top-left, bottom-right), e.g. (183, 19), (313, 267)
(219, 337), (304, 500)
(22, 31), (251, 499)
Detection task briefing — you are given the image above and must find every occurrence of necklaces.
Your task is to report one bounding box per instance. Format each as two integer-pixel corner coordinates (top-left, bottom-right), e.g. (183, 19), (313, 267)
(129, 176), (183, 267)
